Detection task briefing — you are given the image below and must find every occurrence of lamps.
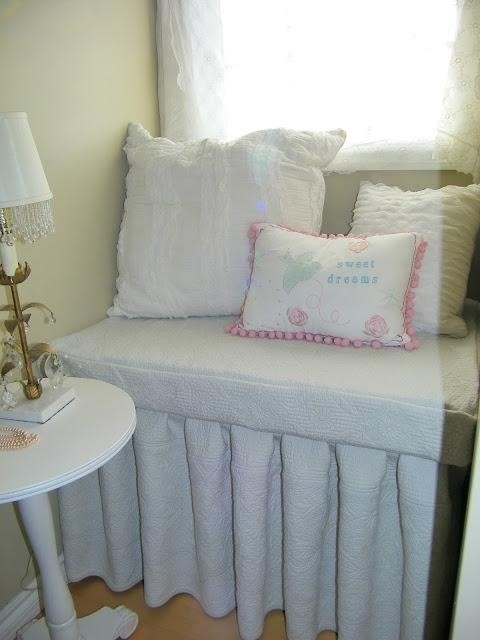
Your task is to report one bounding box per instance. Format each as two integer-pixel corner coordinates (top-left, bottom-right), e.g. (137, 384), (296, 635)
(0, 107), (78, 426)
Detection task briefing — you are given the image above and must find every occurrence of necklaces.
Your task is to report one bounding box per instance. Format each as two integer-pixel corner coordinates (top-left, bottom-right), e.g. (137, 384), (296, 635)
(0, 423), (40, 450)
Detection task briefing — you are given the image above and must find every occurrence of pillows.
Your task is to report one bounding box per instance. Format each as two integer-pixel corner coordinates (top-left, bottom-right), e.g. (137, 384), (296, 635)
(225, 223), (430, 352)
(107, 120), (346, 319)
(346, 180), (479, 338)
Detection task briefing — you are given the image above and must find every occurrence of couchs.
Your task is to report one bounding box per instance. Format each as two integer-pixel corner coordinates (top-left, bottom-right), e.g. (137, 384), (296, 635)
(49, 168), (479, 637)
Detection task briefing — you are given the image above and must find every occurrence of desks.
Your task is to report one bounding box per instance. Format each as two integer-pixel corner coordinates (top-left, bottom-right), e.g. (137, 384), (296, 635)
(0, 374), (150, 639)
